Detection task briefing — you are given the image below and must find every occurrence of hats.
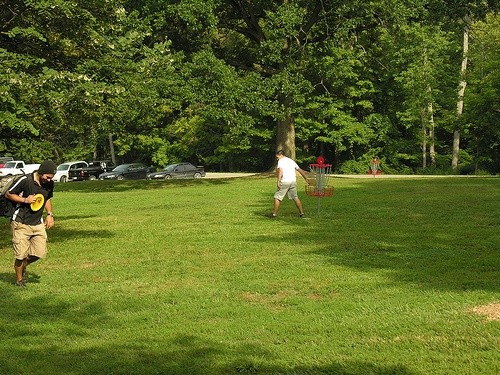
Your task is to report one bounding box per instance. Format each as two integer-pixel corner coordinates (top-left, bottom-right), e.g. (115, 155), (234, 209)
(39, 160), (58, 173)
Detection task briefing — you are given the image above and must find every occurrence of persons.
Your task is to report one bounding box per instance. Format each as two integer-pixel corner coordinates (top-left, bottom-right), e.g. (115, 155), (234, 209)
(265, 150), (308, 218)
(5, 161), (57, 285)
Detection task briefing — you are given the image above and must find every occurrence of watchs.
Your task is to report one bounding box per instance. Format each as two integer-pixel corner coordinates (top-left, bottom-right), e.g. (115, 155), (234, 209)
(48, 212), (54, 216)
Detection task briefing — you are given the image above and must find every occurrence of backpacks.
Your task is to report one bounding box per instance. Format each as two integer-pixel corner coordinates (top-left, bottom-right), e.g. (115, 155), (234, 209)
(0, 168), (34, 218)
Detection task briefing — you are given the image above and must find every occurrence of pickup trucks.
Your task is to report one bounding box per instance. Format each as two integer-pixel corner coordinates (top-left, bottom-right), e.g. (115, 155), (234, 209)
(0, 160), (42, 180)
(68, 160), (118, 183)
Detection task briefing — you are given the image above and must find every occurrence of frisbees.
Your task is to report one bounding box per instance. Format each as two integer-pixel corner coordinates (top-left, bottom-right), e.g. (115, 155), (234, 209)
(30, 194), (44, 211)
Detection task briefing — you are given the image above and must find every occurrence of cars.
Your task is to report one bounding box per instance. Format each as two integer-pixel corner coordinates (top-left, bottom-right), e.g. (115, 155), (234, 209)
(51, 160), (90, 183)
(99, 163), (155, 183)
(0, 157), (14, 164)
(147, 162), (206, 180)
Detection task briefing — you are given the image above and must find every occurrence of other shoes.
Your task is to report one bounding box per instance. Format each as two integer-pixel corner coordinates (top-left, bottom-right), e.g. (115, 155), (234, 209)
(17, 280), (26, 287)
(21, 270), (27, 283)
(299, 213), (305, 218)
(268, 214), (277, 218)
